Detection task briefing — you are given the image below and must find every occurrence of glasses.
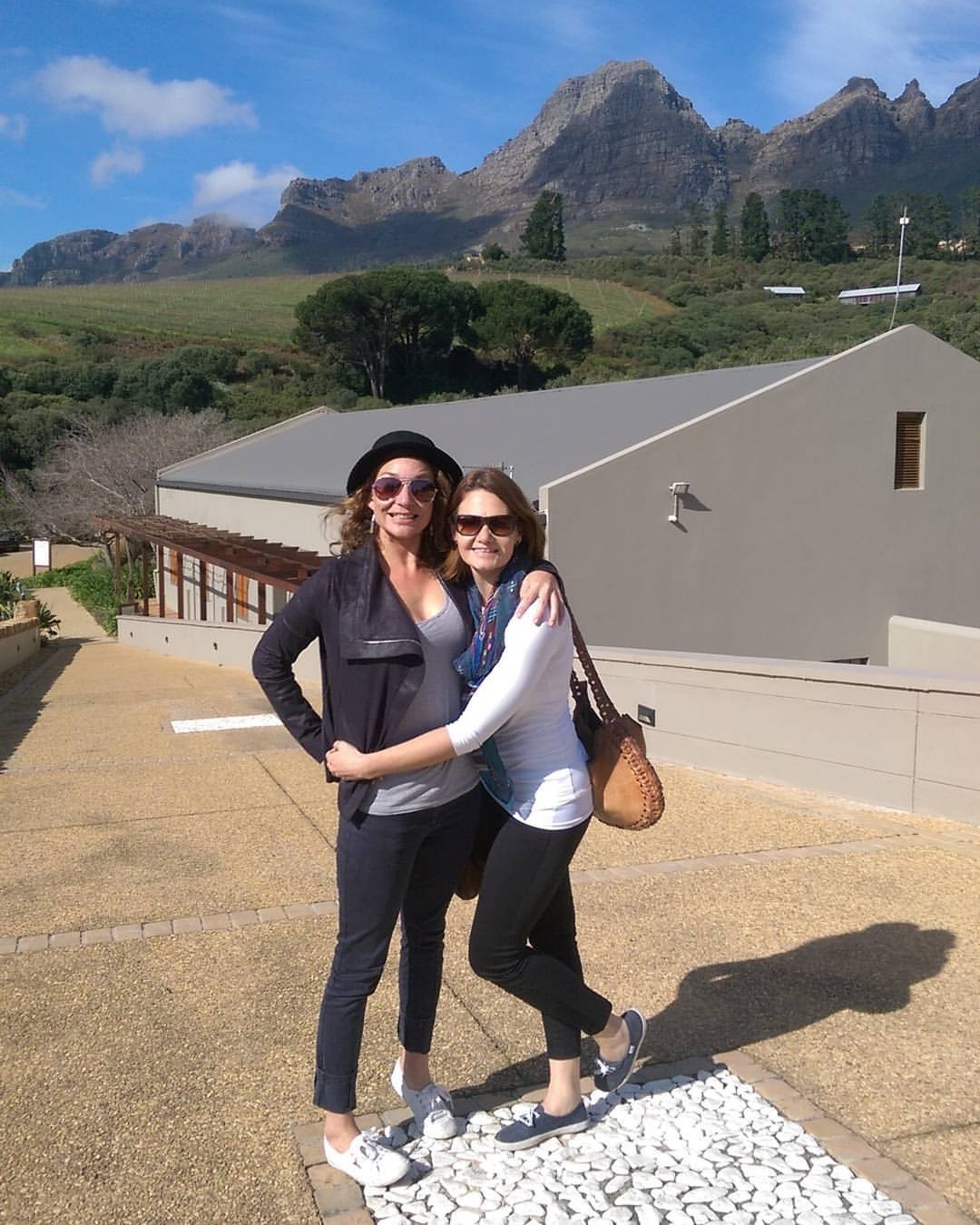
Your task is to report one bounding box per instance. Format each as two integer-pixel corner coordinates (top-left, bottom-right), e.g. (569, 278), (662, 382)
(369, 477), (439, 503)
(454, 513), (518, 537)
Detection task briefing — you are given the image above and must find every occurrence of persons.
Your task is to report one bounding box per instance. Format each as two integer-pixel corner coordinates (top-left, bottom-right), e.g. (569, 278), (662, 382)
(325, 462), (648, 1152)
(253, 429), (566, 1189)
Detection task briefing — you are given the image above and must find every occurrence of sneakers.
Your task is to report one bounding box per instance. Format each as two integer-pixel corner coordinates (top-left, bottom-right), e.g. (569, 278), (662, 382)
(495, 1098), (592, 1151)
(593, 1008), (647, 1093)
(390, 1056), (458, 1139)
(322, 1125), (413, 1187)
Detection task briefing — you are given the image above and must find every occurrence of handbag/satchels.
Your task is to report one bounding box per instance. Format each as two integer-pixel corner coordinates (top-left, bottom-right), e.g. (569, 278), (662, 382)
(563, 593), (665, 831)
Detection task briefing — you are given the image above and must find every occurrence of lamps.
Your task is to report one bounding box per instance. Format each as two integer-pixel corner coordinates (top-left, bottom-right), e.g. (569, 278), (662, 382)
(668, 482), (689, 524)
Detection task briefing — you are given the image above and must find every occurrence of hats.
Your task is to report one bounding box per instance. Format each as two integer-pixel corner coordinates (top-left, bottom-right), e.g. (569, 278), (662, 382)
(346, 430), (463, 497)
